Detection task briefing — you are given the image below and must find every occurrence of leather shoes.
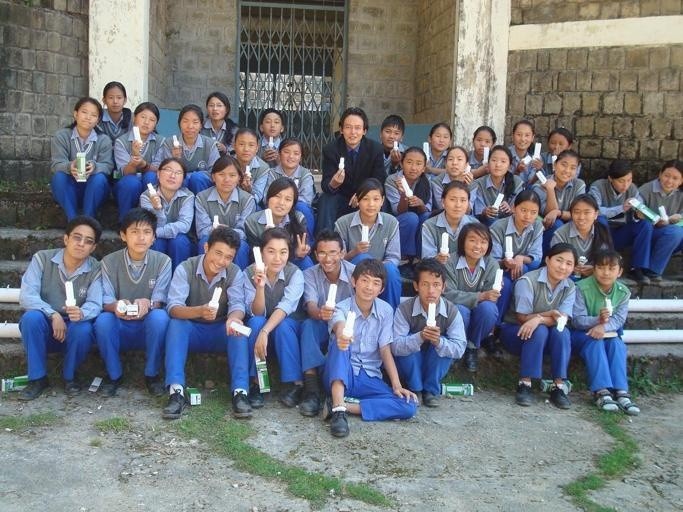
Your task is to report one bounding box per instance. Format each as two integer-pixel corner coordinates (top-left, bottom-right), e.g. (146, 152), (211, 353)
(276, 381), (304, 408)
(643, 268), (662, 281)
(248, 382), (265, 408)
(462, 346), (479, 372)
(515, 381), (533, 406)
(321, 393), (334, 421)
(100, 377), (123, 398)
(627, 266), (651, 286)
(414, 391), (424, 408)
(160, 387), (188, 419)
(63, 379), (82, 393)
(297, 381), (322, 417)
(329, 406), (350, 437)
(421, 390), (440, 407)
(143, 375), (165, 396)
(550, 382), (572, 409)
(482, 334), (504, 358)
(17, 375), (50, 402)
(230, 391), (254, 418)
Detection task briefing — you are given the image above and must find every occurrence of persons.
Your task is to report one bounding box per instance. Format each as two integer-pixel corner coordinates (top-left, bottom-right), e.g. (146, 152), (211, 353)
(331, 178), (400, 311)
(474, 146), (528, 228)
(300, 229), (356, 417)
(547, 195), (611, 266)
(464, 124), (497, 181)
(316, 257), (419, 438)
(14, 217), (104, 400)
(385, 146), (435, 284)
(503, 120), (542, 192)
(538, 127), (584, 183)
(376, 114), (412, 177)
(441, 222), (504, 373)
(242, 176), (314, 271)
(193, 154), (256, 273)
(114, 102), (166, 226)
(261, 138), (316, 235)
(198, 90), (240, 156)
(241, 227), (304, 408)
(634, 160), (683, 284)
(48, 96), (112, 224)
(160, 104), (222, 198)
(588, 159), (653, 290)
(228, 127), (268, 209)
(497, 240), (577, 409)
(95, 82), (134, 146)
(417, 122), (452, 184)
(489, 190), (545, 328)
(532, 150), (587, 240)
(428, 147), (478, 220)
(159, 224), (253, 419)
(94, 208), (173, 399)
(391, 256), (467, 408)
(255, 106), (290, 170)
(316, 106), (386, 239)
(421, 180), (482, 268)
(573, 250), (642, 416)
(138, 158), (196, 276)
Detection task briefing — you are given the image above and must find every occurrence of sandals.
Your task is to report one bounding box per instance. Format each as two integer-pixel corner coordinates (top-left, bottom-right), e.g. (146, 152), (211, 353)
(592, 389), (620, 412)
(613, 391), (641, 416)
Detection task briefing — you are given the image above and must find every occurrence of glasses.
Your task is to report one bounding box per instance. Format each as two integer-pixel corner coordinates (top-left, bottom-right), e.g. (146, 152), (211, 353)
(314, 250), (341, 258)
(420, 280), (442, 289)
(549, 256), (574, 268)
(517, 208), (539, 216)
(160, 167), (184, 177)
(69, 233), (95, 245)
